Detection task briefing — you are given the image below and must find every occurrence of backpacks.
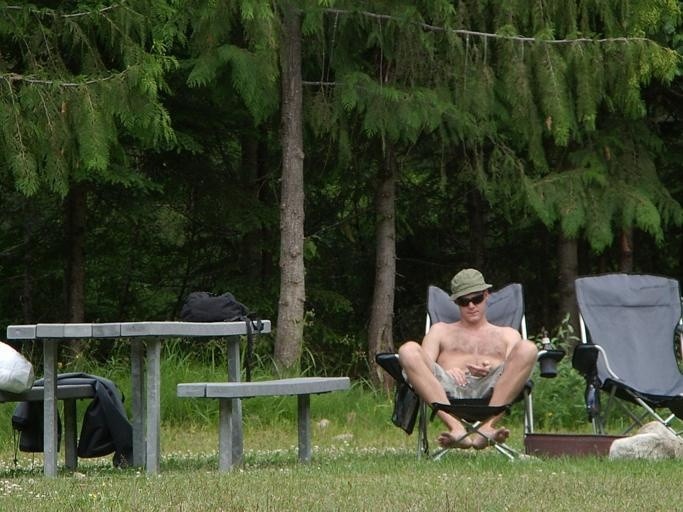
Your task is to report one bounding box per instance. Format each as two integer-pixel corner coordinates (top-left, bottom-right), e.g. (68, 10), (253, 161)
(181, 291), (256, 322)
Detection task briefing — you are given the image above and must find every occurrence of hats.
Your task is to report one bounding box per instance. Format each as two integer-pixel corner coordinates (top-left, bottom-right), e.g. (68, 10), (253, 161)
(449, 269), (493, 300)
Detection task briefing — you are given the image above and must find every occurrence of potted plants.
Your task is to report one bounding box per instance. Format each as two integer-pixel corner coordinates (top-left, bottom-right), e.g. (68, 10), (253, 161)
(528, 312), (580, 377)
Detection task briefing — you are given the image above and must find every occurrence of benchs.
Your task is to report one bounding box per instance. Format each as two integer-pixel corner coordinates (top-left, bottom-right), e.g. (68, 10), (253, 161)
(177, 377), (350, 475)
(1, 386), (96, 470)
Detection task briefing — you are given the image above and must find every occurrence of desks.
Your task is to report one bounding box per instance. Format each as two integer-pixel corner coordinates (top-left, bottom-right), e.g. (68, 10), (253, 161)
(6, 320), (271, 477)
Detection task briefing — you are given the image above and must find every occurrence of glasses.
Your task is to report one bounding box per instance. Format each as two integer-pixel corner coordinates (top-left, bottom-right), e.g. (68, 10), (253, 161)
(456, 294), (484, 305)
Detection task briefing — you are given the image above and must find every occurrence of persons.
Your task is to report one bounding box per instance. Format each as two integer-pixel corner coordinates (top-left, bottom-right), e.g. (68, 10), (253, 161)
(399, 268), (538, 449)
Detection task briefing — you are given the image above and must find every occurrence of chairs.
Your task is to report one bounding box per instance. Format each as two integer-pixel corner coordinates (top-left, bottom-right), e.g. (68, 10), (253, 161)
(572, 274), (683, 440)
(376, 283), (565, 461)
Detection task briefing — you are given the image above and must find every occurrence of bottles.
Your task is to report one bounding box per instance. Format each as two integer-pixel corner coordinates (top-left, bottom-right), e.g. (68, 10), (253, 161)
(539, 331), (558, 378)
